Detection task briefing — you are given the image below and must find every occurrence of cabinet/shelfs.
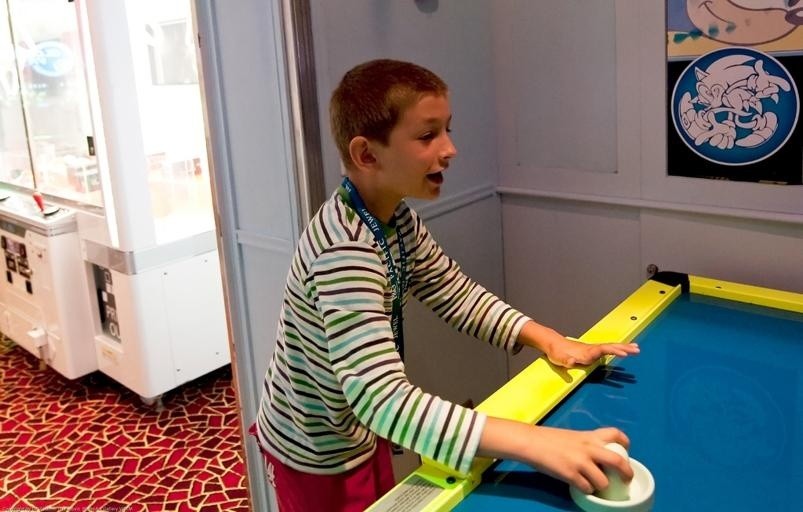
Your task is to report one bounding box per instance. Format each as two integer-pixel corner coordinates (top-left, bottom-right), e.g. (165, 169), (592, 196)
(2, 1), (231, 408)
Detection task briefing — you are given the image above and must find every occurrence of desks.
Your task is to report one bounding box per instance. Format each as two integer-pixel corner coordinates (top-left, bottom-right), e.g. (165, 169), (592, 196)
(363, 271), (803, 512)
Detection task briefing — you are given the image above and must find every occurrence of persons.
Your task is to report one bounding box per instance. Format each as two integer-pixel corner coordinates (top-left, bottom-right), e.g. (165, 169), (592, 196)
(249, 59), (640, 511)
